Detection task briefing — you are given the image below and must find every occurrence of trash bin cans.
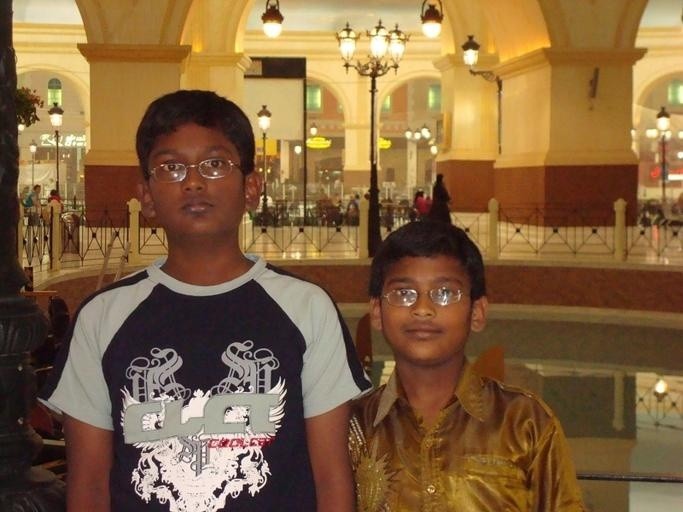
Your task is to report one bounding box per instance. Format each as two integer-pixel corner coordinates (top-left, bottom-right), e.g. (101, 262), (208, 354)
(60, 212), (79, 254)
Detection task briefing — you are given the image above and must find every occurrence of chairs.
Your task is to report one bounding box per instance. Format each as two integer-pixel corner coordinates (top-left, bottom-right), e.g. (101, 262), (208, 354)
(248, 199), (412, 225)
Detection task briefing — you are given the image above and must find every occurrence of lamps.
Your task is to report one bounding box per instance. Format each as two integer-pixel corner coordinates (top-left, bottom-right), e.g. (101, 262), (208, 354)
(420, 0), (444, 38)
(461, 35), (481, 65)
(294, 123), (438, 155)
(262, 0), (284, 38)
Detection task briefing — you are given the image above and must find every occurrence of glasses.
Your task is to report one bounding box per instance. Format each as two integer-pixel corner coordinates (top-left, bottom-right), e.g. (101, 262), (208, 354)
(148, 158), (241, 184)
(380, 286), (463, 306)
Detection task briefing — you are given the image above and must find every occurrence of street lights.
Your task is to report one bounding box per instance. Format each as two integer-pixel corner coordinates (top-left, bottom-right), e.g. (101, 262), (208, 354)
(28, 138), (37, 185)
(46, 99), (63, 199)
(336, 20), (411, 257)
(655, 105), (672, 224)
(257, 104), (271, 229)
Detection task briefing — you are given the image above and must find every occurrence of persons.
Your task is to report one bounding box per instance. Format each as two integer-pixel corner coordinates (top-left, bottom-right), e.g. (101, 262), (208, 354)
(45, 90), (376, 511)
(21, 184), (64, 212)
(346, 217), (585, 512)
(413, 174), (451, 223)
